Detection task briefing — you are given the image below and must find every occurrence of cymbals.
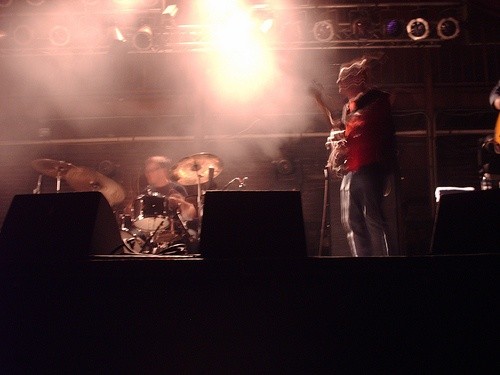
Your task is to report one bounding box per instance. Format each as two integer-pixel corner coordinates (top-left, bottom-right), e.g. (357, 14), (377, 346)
(30, 158), (73, 178)
(64, 164), (126, 206)
(168, 153), (225, 186)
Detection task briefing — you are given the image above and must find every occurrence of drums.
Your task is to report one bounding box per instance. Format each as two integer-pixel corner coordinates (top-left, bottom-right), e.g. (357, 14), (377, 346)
(119, 214), (132, 232)
(130, 193), (172, 233)
(149, 211), (187, 244)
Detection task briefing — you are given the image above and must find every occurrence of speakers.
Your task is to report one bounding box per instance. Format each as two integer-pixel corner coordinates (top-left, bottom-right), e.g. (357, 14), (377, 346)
(429, 187), (500, 255)
(0, 191), (124, 259)
(199, 190), (307, 258)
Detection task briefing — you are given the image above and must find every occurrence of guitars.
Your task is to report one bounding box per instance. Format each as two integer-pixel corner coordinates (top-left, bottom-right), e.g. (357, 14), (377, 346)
(305, 84), (347, 178)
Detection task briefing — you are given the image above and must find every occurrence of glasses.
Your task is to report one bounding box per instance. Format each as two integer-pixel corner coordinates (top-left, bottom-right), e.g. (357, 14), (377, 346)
(146, 167), (161, 172)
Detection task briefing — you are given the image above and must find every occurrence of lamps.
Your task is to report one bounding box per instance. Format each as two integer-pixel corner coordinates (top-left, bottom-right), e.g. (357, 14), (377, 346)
(437, 17), (460, 39)
(407, 17), (430, 40)
(313, 20), (338, 42)
(133, 26), (153, 51)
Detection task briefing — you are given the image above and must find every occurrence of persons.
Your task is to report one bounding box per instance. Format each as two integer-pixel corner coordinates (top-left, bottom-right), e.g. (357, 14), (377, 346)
(124, 156), (190, 213)
(330, 55), (403, 256)
(490, 81), (500, 144)
